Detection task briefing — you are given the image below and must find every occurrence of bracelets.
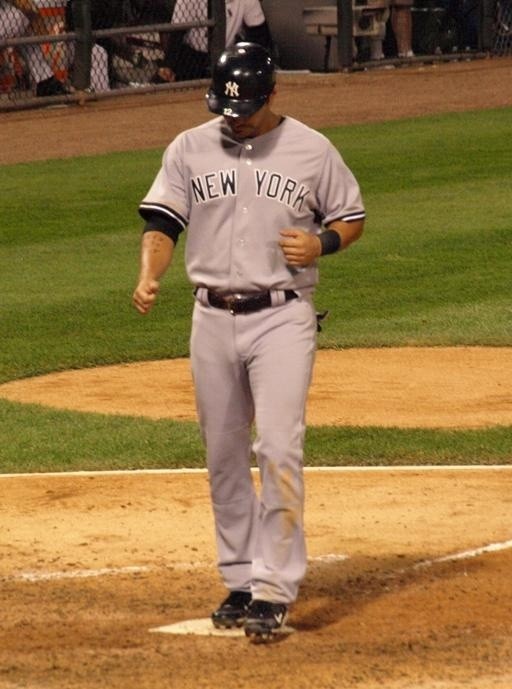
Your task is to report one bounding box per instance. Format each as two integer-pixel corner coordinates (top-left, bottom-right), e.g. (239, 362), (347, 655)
(316, 229), (341, 257)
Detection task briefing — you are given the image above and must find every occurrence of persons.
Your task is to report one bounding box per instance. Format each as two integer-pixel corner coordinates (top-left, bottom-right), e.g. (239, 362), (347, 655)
(131, 41), (367, 636)
(367, 0), (415, 59)
(1, 0), (278, 101)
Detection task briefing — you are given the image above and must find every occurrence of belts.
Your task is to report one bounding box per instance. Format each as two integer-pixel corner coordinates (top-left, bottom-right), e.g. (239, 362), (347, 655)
(192, 284), (298, 316)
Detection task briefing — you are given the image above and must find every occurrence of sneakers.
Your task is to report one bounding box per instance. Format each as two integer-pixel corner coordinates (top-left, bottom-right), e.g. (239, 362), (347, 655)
(212, 591), (252, 629)
(244, 600), (287, 641)
(37, 80), (70, 96)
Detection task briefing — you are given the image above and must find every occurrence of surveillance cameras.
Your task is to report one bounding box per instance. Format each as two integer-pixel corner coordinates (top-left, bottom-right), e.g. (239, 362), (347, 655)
(303, 5), (390, 37)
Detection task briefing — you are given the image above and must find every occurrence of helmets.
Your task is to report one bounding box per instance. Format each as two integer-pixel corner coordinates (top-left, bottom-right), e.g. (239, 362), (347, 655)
(206, 42), (276, 120)
